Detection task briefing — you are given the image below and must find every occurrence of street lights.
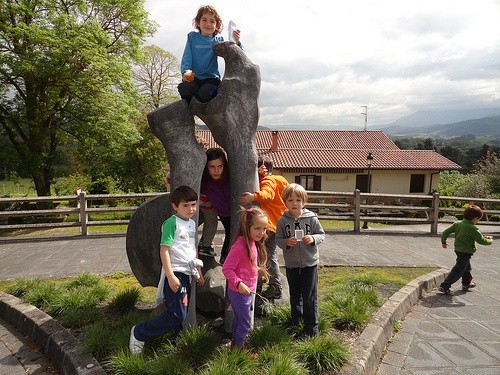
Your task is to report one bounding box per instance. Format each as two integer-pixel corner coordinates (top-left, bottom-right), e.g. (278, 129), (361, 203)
(362, 152), (374, 230)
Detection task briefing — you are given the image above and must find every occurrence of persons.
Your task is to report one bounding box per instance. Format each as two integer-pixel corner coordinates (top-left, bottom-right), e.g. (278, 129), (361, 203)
(275, 183), (326, 341)
(176, 4), (244, 102)
(166, 136), (230, 266)
(240, 156), (289, 299)
(436, 205), (493, 295)
(128, 186), (205, 355)
(222, 206), (271, 358)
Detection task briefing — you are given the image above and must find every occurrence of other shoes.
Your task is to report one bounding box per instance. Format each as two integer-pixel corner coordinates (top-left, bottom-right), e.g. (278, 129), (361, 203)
(199, 239), (214, 246)
(129, 326), (144, 353)
(462, 282), (469, 287)
(231, 343), (248, 348)
(298, 333), (309, 339)
(256, 279), (265, 293)
(286, 324), (296, 333)
(263, 286), (281, 299)
(199, 246), (217, 256)
(438, 287), (455, 295)
